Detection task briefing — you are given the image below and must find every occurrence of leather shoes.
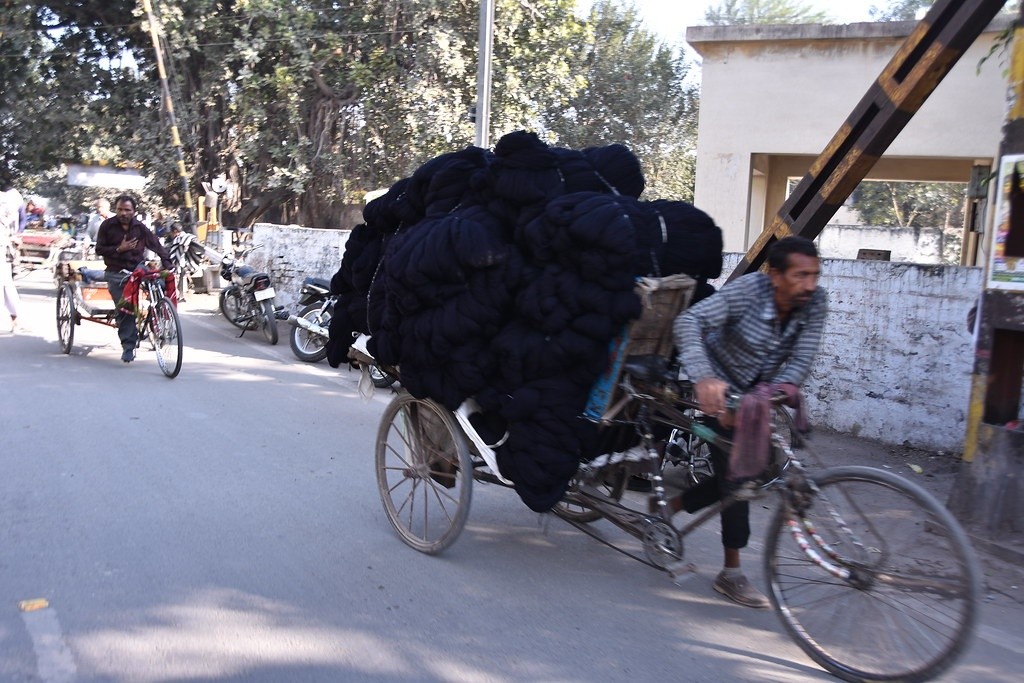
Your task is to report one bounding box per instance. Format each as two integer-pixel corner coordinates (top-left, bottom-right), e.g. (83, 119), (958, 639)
(712, 572), (771, 609)
(649, 495), (669, 522)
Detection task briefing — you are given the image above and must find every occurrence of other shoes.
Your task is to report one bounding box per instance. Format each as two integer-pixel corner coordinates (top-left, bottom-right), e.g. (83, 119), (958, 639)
(122, 349), (134, 362)
(11, 317), (23, 334)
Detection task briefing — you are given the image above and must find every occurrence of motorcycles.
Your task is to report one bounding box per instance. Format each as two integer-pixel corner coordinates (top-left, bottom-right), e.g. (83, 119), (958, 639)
(287, 277), (398, 388)
(218, 244), (289, 346)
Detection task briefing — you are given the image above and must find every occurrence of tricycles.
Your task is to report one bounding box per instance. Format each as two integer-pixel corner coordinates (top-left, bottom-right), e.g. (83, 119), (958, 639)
(56, 267), (183, 379)
(375, 384), (983, 682)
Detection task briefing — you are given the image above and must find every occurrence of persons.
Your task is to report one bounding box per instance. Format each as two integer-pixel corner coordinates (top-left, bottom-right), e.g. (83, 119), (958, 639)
(0, 177), (27, 332)
(650, 236), (829, 606)
(86, 196), (178, 360)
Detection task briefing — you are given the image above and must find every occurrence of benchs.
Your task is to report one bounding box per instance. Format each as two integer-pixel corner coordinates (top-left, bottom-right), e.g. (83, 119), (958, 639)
(20, 229), (67, 266)
(58, 259), (150, 317)
